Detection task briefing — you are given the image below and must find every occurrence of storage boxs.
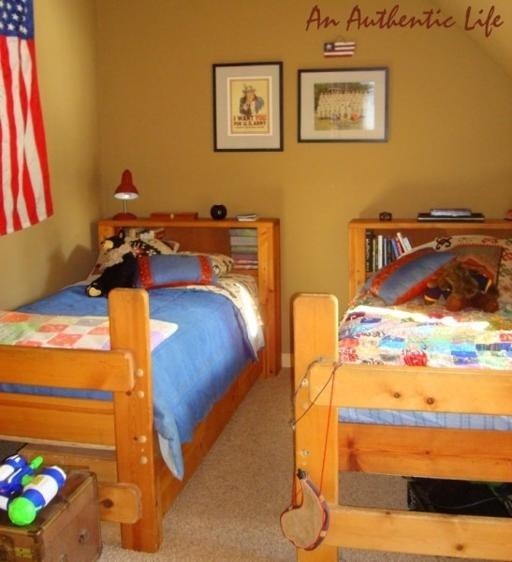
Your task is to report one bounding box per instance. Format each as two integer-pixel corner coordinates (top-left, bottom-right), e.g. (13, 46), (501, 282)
(0, 460), (103, 562)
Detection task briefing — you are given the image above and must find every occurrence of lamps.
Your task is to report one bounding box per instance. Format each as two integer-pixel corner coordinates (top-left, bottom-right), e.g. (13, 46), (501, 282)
(111, 169), (141, 221)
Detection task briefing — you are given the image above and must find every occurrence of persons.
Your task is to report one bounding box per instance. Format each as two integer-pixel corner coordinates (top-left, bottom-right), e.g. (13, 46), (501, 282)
(236, 86), (263, 124)
(316, 86), (372, 120)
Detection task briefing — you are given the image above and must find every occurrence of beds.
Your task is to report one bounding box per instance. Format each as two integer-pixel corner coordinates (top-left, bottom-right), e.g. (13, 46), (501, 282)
(279, 202), (510, 559)
(1, 213), (278, 556)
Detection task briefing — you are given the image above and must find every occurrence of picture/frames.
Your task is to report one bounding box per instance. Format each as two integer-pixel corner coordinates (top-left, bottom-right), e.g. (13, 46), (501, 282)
(211, 60), (286, 154)
(293, 65), (390, 145)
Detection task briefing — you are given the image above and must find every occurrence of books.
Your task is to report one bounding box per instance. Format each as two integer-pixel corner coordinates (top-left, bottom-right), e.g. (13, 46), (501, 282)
(235, 213), (259, 222)
(365, 231), (413, 273)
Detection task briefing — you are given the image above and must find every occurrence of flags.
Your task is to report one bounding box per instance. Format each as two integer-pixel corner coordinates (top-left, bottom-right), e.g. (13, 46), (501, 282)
(0, 1), (53, 235)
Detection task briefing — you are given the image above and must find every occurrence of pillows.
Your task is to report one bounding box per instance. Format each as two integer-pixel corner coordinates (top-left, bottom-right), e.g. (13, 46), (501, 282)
(131, 252), (215, 288)
(176, 251), (235, 277)
(361, 246), (457, 308)
(85, 233), (179, 279)
(448, 238), (506, 294)
